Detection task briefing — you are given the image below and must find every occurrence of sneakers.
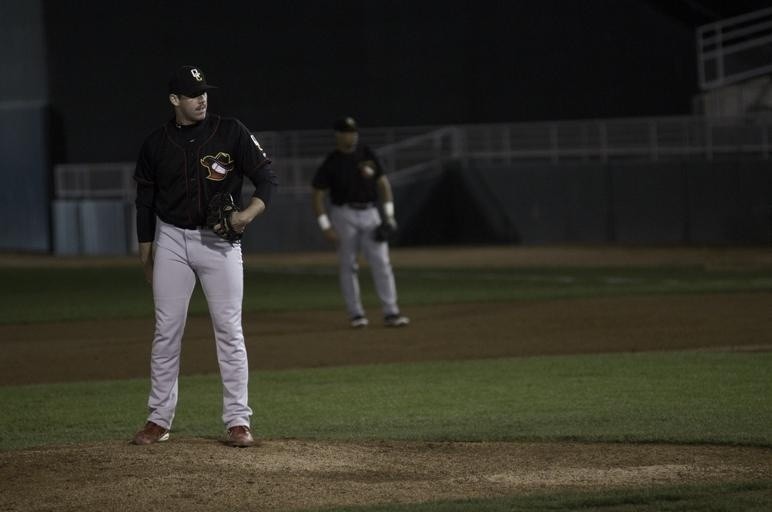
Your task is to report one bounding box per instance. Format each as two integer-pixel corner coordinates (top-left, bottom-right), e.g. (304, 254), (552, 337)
(349, 316), (370, 330)
(383, 314), (411, 329)
(130, 422), (171, 445)
(225, 427), (256, 448)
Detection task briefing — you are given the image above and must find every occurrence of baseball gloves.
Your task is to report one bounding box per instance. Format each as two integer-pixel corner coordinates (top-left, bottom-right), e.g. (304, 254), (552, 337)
(207, 191), (245, 243)
(375, 219), (398, 240)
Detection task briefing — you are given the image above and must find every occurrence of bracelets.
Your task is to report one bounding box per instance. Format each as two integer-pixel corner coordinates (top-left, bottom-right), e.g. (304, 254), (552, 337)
(316, 213), (332, 232)
(382, 200), (395, 218)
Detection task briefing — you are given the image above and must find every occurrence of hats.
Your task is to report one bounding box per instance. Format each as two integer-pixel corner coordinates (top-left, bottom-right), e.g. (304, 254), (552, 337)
(168, 64), (220, 96)
(332, 114), (362, 133)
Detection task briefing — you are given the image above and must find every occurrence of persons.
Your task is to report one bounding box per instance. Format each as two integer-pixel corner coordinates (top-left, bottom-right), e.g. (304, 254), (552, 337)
(127, 58), (275, 448)
(310, 114), (411, 328)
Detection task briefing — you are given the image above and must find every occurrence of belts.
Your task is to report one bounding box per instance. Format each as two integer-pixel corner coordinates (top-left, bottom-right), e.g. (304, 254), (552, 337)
(345, 202), (376, 210)
